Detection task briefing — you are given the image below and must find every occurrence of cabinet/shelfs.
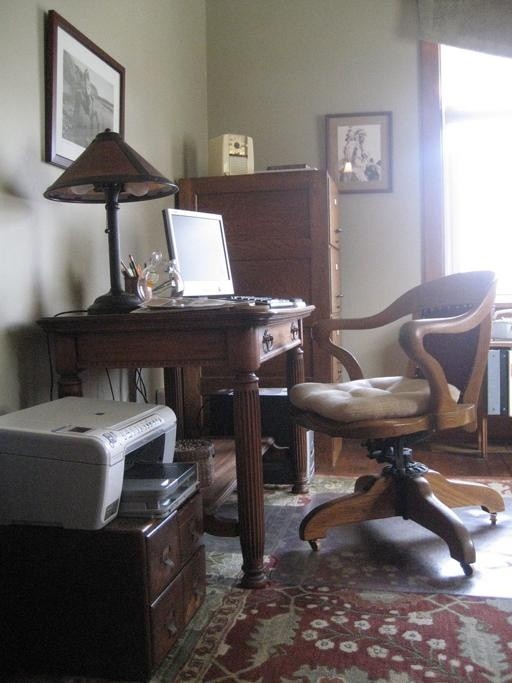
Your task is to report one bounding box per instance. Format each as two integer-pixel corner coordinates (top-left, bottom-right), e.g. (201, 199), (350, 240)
(178, 170), (342, 469)
(0, 496), (207, 683)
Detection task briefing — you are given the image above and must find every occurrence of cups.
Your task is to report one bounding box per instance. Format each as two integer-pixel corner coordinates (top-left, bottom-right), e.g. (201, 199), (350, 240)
(125, 277), (139, 296)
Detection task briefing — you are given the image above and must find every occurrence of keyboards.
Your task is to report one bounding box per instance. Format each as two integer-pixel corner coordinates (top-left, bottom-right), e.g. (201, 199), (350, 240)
(227, 293), (296, 311)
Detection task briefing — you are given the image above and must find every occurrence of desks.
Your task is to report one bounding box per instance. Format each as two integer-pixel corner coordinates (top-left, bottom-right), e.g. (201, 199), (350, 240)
(38, 303), (316, 592)
(477, 339), (512, 457)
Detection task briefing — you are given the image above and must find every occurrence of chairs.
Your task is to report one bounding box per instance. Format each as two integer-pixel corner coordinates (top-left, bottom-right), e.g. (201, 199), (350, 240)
(292, 271), (502, 577)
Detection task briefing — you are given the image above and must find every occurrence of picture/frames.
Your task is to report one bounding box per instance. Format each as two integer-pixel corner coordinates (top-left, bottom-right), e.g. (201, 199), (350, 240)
(43, 11), (127, 170)
(325, 112), (392, 194)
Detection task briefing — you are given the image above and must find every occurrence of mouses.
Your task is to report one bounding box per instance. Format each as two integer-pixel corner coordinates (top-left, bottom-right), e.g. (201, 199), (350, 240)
(289, 296), (306, 311)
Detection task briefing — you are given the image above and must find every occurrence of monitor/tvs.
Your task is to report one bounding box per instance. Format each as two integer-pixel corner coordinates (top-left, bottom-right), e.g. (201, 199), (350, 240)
(161, 207), (237, 303)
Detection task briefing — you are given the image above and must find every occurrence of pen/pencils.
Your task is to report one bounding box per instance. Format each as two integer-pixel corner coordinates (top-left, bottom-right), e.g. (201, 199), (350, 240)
(119, 254), (141, 278)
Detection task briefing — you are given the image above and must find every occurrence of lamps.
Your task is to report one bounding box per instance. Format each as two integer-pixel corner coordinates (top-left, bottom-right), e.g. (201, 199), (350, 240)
(44, 129), (178, 315)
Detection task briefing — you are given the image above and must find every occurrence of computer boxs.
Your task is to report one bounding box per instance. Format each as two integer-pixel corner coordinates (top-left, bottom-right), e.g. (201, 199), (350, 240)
(203, 386), (316, 485)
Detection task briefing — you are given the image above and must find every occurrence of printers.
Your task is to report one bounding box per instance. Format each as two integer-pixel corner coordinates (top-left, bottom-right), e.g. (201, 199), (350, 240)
(1, 395), (201, 530)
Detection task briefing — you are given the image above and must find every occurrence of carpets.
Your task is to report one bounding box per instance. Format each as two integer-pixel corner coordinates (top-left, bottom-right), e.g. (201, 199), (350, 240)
(147, 475), (512, 683)
